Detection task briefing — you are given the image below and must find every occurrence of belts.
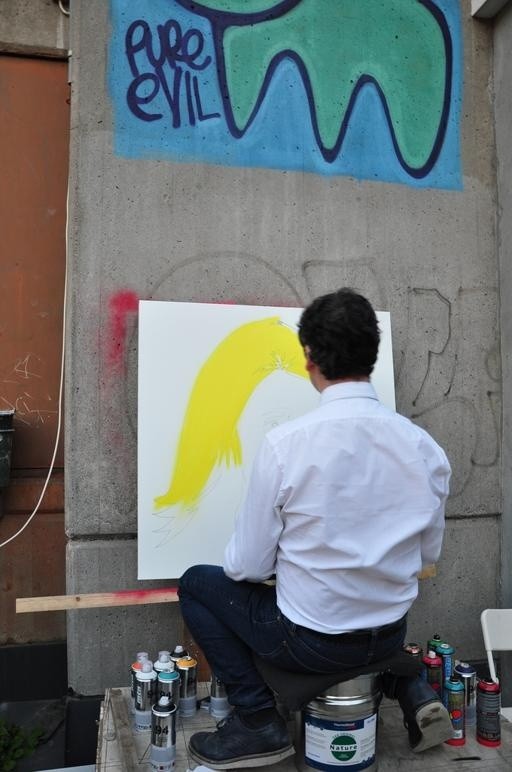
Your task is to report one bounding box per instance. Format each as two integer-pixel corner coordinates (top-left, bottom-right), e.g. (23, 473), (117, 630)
(300, 611), (408, 643)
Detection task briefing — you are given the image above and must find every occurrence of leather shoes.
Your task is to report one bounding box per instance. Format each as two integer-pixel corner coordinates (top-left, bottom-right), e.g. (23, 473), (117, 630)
(187, 708), (296, 770)
(397, 679), (454, 753)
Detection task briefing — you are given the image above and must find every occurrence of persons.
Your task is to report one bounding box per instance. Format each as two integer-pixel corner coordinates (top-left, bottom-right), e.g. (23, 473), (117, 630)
(179, 287), (458, 769)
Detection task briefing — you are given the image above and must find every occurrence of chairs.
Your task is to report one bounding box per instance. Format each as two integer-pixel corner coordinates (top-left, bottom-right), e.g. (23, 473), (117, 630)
(481, 610), (512, 724)
